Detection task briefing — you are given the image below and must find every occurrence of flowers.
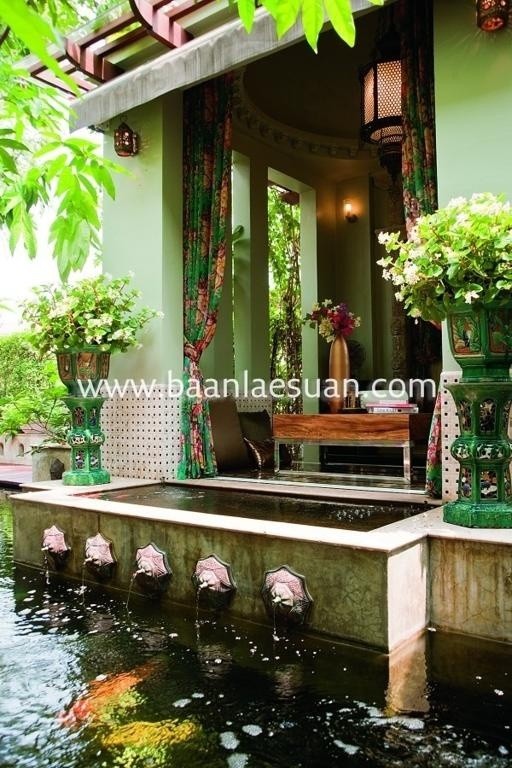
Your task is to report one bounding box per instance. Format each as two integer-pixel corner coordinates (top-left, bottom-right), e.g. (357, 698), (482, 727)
(303, 296), (363, 342)
(16, 270), (167, 364)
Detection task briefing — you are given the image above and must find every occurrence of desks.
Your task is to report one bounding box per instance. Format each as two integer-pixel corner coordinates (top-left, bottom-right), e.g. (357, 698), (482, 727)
(270, 413), (434, 486)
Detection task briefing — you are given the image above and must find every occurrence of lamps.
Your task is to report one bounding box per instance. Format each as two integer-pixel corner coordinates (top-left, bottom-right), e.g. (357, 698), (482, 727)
(112, 114), (140, 157)
(476, 1), (510, 33)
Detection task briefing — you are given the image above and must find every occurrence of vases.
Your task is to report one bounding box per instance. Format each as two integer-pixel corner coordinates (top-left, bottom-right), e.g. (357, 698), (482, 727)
(434, 310), (510, 529)
(41, 345), (110, 486)
(376, 186), (510, 326)
(327, 330), (351, 413)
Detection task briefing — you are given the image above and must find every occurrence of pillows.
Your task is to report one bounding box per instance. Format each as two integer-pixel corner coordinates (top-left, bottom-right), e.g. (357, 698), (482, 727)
(243, 436), (288, 470)
